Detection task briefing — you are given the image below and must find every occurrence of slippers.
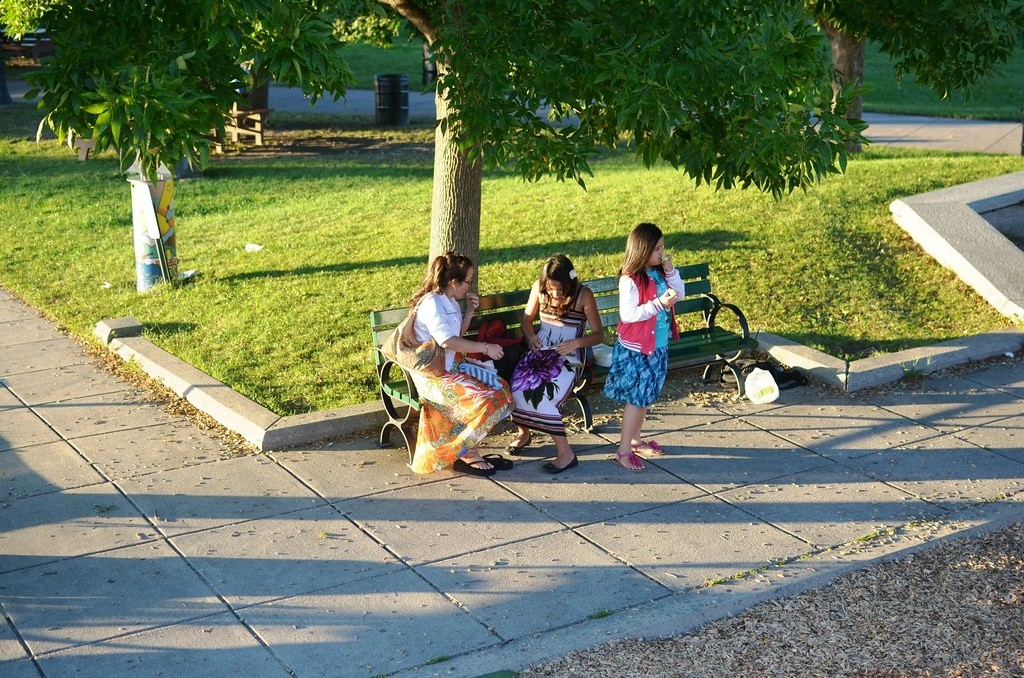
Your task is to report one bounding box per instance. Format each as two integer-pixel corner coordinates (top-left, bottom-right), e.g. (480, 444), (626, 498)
(454, 457), (496, 477)
(483, 453), (514, 471)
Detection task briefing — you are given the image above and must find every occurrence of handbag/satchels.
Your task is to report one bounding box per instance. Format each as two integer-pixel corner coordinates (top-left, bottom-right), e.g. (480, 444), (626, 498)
(718, 358), (808, 390)
(470, 318), (528, 384)
(379, 290), (445, 376)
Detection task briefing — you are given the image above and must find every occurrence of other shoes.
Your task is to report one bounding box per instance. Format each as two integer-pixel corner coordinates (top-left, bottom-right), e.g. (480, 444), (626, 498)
(504, 432), (532, 456)
(543, 452), (579, 473)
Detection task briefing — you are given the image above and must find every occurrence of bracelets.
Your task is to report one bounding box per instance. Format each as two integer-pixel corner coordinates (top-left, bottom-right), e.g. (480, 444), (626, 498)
(483, 342), (488, 355)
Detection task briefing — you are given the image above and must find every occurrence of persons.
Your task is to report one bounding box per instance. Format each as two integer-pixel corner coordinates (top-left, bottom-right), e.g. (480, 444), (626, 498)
(406, 251), (516, 476)
(602, 223), (686, 472)
(507, 254), (604, 473)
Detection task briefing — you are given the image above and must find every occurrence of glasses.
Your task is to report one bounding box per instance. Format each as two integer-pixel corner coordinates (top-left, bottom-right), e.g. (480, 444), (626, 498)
(460, 279), (473, 289)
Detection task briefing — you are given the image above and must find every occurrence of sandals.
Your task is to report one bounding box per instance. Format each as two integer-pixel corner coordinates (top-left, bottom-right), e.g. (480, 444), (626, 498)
(631, 439), (667, 455)
(611, 450), (647, 472)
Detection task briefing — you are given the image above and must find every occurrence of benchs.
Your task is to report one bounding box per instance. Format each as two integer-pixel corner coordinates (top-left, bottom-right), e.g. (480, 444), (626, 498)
(371, 262), (759, 465)
